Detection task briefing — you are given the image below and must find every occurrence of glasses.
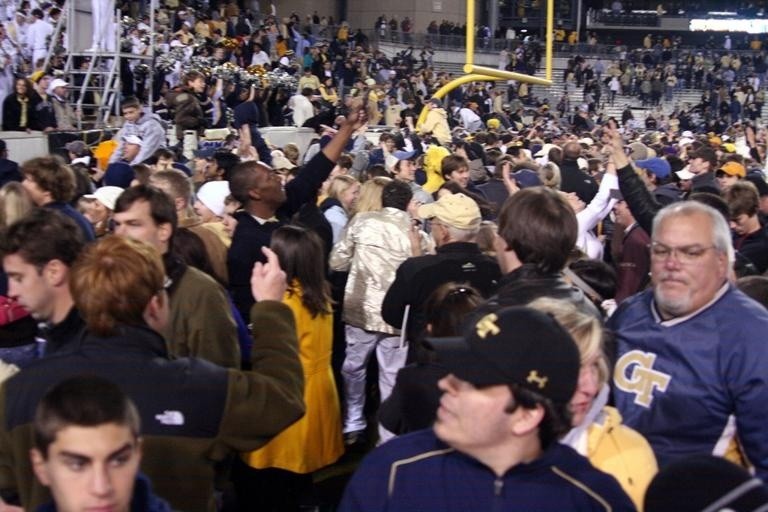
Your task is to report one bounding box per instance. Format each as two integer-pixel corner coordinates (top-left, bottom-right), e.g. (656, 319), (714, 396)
(425, 220), (448, 228)
(645, 243), (716, 260)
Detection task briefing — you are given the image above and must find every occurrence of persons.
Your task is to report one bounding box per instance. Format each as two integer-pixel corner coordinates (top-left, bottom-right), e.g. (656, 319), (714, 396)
(201, 148), (240, 213)
(192, 180), (231, 246)
(113, 182), (239, 371)
(221, 192), (242, 241)
(148, 147), (173, 170)
(108, 97), (168, 166)
(64, 142), (94, 171)
(675, 164), (696, 199)
(605, 201), (768, 512)
(380, 193), (504, 366)
(0, 137), (19, 184)
(0, 235), (307, 512)
(553, 155), (619, 262)
(0, 209), (87, 373)
(2, 77), (39, 133)
(339, 306), (639, 512)
(0, 180), (33, 235)
(228, 103), (369, 315)
(239, 224), (346, 475)
(21, 156), (98, 243)
(32, 69), (58, 134)
(684, 147), (720, 195)
(328, 179), (433, 447)
(480, 186), (604, 324)
(121, 133), (142, 162)
(522, 296), (658, 512)
(119, 1), (768, 137)
(715, 161), (746, 191)
(369, 129), (598, 201)
(85, 186), (125, 235)
(49, 79), (77, 132)
(419, 282), (487, 338)
(604, 118), (729, 239)
(635, 158), (680, 203)
(27, 374), (146, 512)
(0, 0), (67, 76)
(149, 169), (226, 283)
(609, 188), (651, 302)
(720, 181), (768, 275)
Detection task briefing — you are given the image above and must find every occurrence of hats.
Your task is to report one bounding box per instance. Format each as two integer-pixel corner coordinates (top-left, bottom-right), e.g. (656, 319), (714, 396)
(65, 140), (87, 154)
(285, 48), (295, 55)
(421, 305), (581, 404)
(32, 71), (45, 82)
(708, 136), (721, 144)
(192, 147), (215, 162)
(688, 145), (712, 161)
(510, 169), (539, 188)
(534, 143), (560, 157)
(609, 188), (624, 200)
(271, 156), (297, 174)
(678, 137), (694, 146)
(720, 143), (735, 152)
(425, 98), (442, 106)
(634, 158), (670, 178)
(675, 163), (696, 180)
(418, 191), (482, 233)
(83, 184), (124, 210)
(384, 148), (418, 174)
(643, 450), (768, 511)
(121, 133), (144, 146)
(48, 78), (69, 93)
(197, 180), (231, 217)
(682, 131), (693, 138)
(744, 172), (768, 195)
(716, 160), (746, 178)
